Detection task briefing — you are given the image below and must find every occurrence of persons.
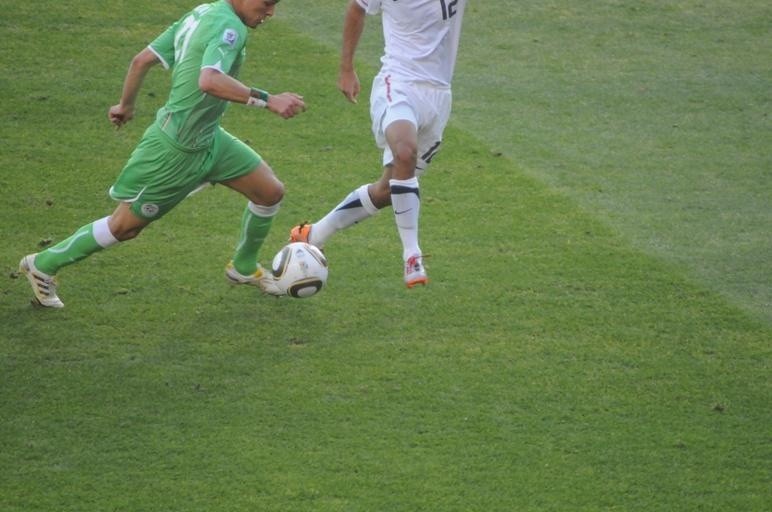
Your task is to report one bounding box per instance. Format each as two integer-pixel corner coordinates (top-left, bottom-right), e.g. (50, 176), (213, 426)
(19, 0), (307, 309)
(288, 1), (469, 288)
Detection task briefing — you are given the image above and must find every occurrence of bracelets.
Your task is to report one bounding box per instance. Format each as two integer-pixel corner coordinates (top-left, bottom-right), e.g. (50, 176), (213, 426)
(246, 87), (269, 109)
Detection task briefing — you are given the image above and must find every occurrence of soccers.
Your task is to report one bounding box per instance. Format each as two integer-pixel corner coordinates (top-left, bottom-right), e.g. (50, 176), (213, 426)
(272, 242), (329, 297)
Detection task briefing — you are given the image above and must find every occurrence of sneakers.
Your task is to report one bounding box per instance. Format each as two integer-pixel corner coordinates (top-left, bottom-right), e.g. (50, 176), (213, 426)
(290, 221), (311, 243)
(404, 254), (427, 288)
(224, 260), (273, 290)
(19, 252), (64, 308)
